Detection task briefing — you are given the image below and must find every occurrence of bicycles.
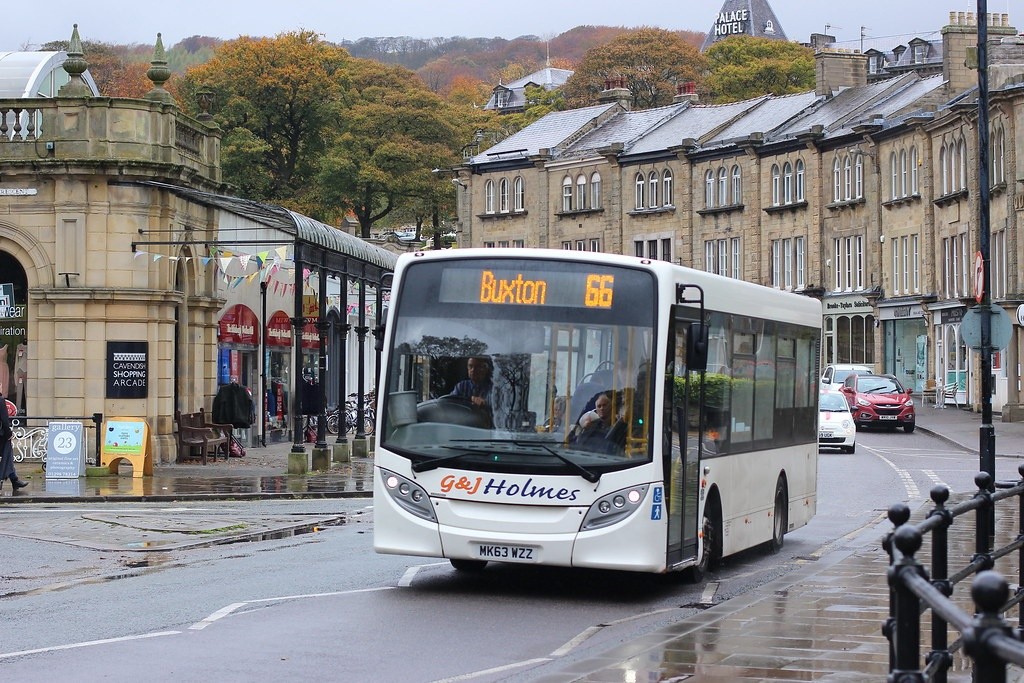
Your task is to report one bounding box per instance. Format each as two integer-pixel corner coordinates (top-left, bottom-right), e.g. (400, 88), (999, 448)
(325, 390), (378, 437)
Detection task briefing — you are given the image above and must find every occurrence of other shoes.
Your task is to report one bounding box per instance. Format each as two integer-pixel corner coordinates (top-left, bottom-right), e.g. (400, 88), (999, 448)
(11, 479), (29, 490)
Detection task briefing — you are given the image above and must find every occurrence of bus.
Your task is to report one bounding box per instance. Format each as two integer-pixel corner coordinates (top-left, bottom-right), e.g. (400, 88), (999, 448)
(371, 247), (825, 585)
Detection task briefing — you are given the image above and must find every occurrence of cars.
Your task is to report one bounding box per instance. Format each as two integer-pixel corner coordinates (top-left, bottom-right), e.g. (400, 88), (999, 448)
(815, 383), (857, 455)
(838, 375), (916, 433)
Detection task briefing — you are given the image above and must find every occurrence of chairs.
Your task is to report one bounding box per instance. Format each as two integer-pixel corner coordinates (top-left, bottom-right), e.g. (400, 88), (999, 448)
(175, 407), (234, 465)
(555, 359), (621, 432)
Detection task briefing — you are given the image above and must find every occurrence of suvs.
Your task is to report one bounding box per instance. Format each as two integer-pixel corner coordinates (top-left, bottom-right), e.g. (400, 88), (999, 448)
(821, 363), (875, 391)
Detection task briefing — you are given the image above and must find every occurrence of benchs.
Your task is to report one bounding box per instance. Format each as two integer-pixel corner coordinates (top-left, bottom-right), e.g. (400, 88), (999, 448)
(920, 381), (959, 409)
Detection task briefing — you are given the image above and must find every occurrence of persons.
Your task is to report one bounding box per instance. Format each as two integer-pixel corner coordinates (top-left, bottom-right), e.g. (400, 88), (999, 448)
(548, 362), (648, 447)
(14, 339), (27, 409)
(0, 342), (10, 398)
(0, 383), (28, 492)
(448, 354), (502, 409)
